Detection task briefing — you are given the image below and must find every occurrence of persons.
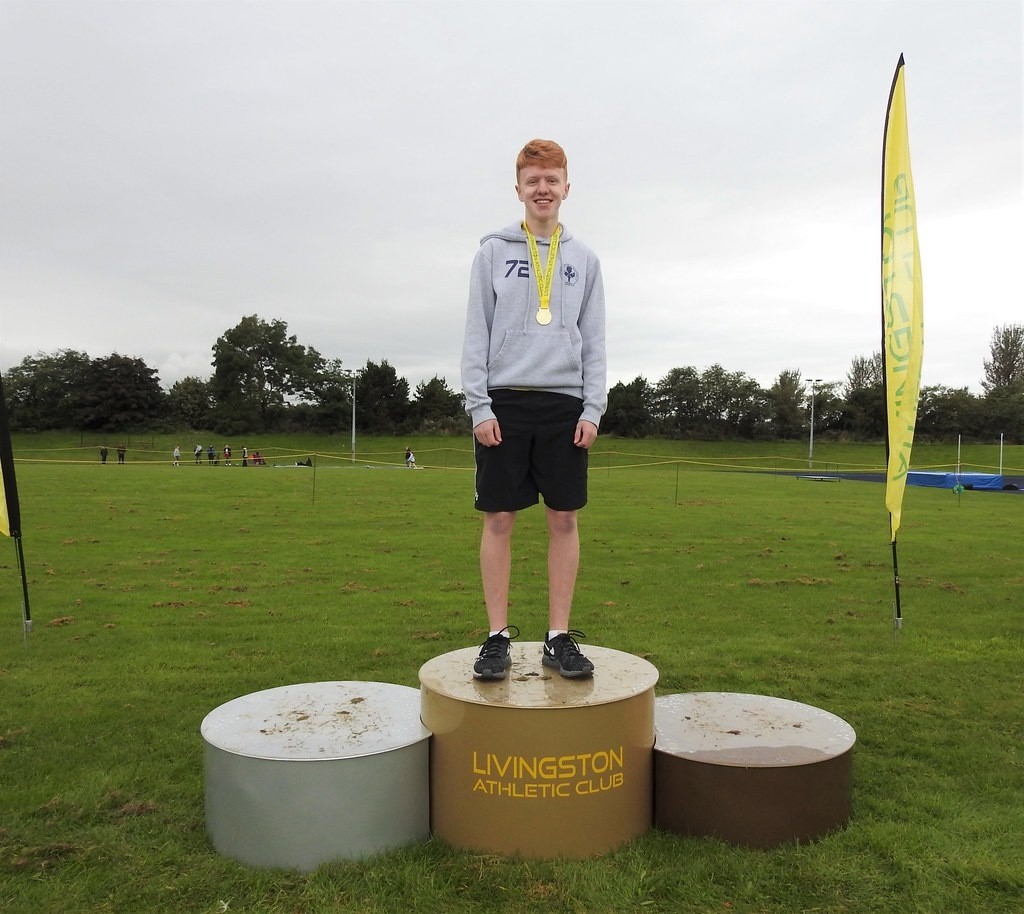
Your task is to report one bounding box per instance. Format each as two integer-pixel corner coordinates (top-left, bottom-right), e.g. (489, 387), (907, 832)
(193, 444), (203, 464)
(224, 445), (232, 465)
(100, 446), (108, 464)
(295, 458), (312, 467)
(407, 452), (417, 468)
(206, 444), (215, 464)
(252, 452), (266, 465)
(117, 443), (126, 464)
(242, 446), (248, 467)
(405, 447), (410, 467)
(173, 446), (180, 466)
(460, 140), (608, 680)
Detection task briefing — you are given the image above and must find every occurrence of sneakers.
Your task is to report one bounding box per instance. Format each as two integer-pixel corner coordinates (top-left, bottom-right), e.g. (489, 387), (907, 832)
(473, 625), (520, 680)
(542, 630), (595, 678)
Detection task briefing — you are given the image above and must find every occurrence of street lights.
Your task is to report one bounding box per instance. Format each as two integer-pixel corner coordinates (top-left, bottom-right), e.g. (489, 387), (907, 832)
(805, 379), (823, 467)
(344, 369), (363, 463)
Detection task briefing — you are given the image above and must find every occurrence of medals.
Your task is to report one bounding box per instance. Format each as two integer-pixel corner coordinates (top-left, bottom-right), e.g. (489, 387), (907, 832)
(536, 309), (552, 325)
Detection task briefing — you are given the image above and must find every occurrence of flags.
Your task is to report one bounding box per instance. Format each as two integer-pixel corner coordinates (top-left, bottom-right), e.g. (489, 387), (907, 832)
(882, 65), (923, 542)
